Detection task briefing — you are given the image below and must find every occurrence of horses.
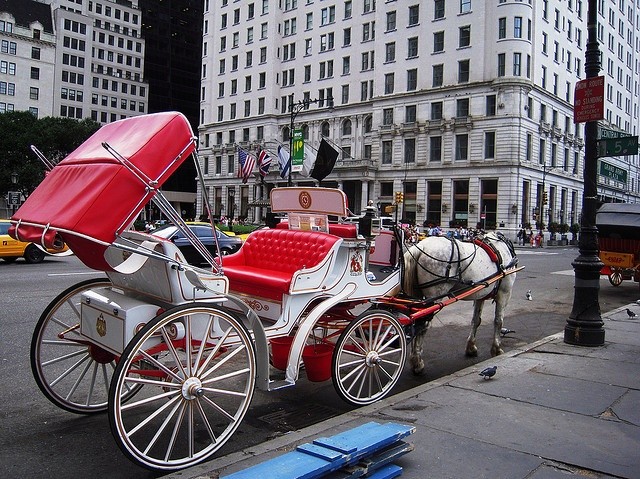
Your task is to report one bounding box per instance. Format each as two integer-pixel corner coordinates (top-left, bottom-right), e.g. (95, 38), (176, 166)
(403, 231), (517, 379)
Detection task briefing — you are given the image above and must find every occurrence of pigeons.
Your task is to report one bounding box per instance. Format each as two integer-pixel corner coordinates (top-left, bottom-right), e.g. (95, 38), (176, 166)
(479, 366), (497, 380)
(625, 308), (639, 320)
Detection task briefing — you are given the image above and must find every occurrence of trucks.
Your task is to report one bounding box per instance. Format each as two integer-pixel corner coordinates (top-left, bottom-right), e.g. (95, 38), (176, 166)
(343, 216), (394, 233)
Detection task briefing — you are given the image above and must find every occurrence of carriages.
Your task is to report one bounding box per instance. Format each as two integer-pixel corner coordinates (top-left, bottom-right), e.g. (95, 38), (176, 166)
(17, 112), (525, 475)
(586, 204), (640, 291)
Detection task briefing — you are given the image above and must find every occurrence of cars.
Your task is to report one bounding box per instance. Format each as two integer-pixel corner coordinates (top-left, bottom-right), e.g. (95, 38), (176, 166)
(150, 223), (244, 268)
(0, 218), (69, 263)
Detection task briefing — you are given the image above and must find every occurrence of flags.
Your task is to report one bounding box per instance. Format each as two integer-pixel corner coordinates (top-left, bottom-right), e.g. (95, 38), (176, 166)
(310, 138), (339, 182)
(277, 142), (290, 178)
(299, 144), (316, 177)
(256, 144), (272, 182)
(237, 147), (255, 184)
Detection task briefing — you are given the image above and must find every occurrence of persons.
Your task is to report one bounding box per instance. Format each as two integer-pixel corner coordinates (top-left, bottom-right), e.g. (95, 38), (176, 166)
(403, 223), (542, 245)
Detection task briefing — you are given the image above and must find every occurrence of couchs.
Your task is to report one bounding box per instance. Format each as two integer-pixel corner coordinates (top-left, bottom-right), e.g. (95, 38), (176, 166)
(213, 229), (343, 295)
(100, 230), (230, 305)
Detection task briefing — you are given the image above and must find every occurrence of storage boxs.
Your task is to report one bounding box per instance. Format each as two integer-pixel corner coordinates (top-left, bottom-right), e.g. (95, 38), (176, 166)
(79, 287), (163, 361)
